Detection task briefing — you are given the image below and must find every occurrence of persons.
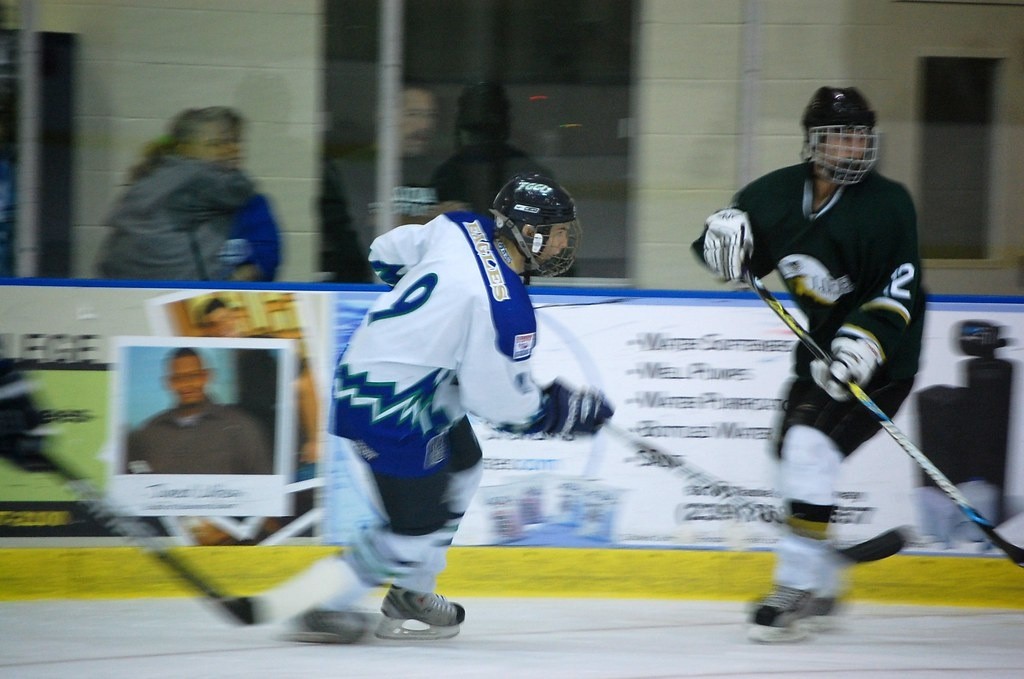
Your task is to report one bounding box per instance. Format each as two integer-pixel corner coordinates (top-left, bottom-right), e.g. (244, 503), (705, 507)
(691, 86), (925, 629)
(95, 106), (282, 281)
(277, 173), (614, 636)
(324, 82), (471, 284)
(125, 348), (270, 472)
(196, 297), (318, 467)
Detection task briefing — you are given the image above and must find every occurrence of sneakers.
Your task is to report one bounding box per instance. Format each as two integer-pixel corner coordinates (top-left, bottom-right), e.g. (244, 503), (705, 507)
(749, 585), (849, 643)
(273, 605), (366, 643)
(374, 585), (465, 640)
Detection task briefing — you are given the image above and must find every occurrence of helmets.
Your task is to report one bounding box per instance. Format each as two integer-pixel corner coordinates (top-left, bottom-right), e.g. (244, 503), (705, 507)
(489, 173), (577, 277)
(803, 87), (876, 181)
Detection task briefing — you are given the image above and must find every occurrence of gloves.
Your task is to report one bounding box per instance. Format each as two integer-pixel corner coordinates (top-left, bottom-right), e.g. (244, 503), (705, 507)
(704, 209), (755, 285)
(808, 324), (884, 401)
(523, 384), (614, 440)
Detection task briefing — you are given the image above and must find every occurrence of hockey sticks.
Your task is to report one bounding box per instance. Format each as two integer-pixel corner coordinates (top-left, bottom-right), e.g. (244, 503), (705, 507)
(44, 456), (354, 630)
(602, 419), (908, 567)
(739, 268), (1024, 569)
